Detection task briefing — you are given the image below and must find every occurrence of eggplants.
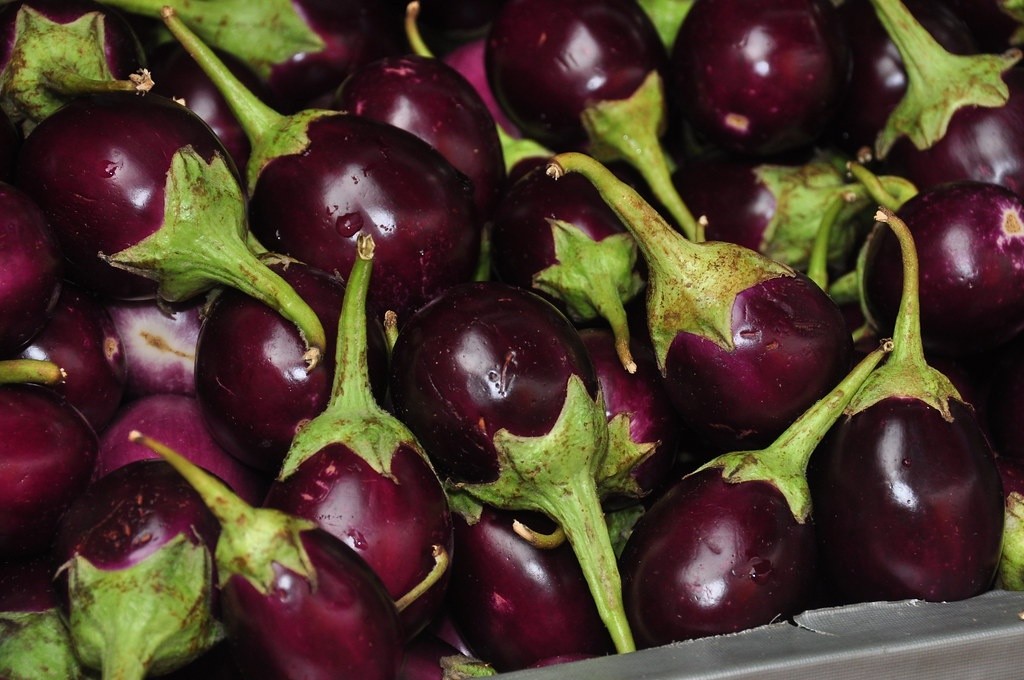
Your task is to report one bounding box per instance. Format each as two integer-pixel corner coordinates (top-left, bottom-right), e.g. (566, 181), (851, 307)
(1, 0), (1023, 680)
